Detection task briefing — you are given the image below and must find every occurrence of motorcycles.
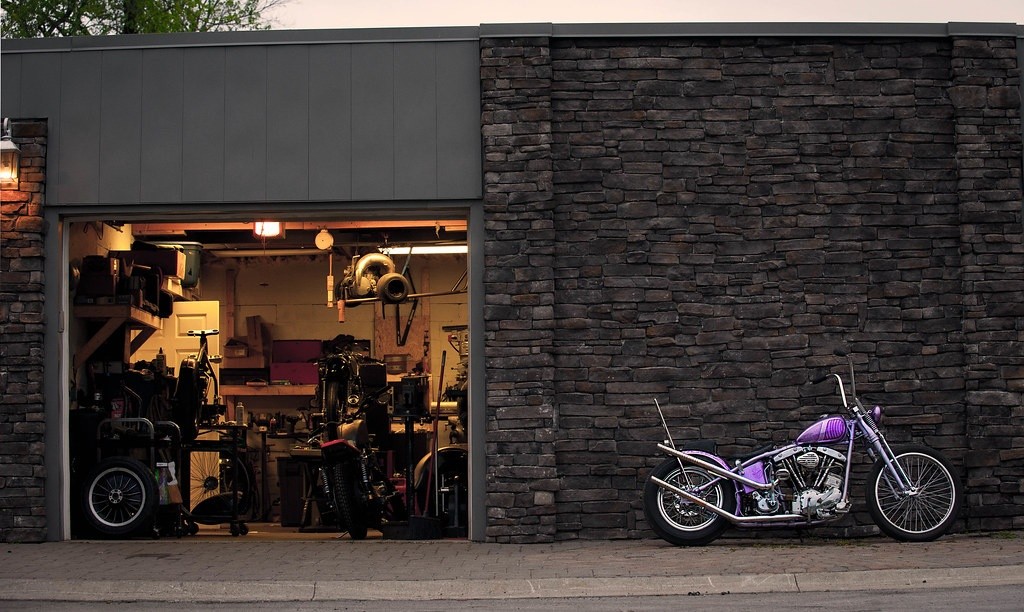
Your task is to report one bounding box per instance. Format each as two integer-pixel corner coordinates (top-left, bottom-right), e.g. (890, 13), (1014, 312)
(306, 375), (409, 540)
(641, 349), (964, 547)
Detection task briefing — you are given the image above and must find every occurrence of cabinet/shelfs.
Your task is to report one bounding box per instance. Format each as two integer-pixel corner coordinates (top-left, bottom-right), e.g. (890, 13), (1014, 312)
(220, 437), (320, 523)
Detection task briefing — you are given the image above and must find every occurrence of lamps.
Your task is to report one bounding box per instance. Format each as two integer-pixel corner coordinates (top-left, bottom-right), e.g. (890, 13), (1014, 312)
(0, 117), (22, 183)
(253, 222), (286, 240)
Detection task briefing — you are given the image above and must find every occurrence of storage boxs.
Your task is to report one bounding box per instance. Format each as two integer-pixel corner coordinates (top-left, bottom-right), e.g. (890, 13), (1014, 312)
(78, 257), (120, 297)
(109, 250), (187, 280)
(223, 345), (249, 358)
(152, 241), (204, 289)
(269, 340), (322, 384)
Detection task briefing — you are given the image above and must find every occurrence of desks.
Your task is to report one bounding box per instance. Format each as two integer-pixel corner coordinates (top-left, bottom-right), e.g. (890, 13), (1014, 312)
(219, 385), (317, 434)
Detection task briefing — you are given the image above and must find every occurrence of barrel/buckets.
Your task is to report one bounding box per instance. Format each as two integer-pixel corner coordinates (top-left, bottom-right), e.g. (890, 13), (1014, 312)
(427, 415), (449, 448)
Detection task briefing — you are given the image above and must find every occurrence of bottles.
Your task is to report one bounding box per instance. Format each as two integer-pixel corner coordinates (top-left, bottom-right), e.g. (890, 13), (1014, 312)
(441, 423), (451, 445)
(270, 417), (277, 435)
(155, 347), (166, 374)
(236, 402), (244, 426)
